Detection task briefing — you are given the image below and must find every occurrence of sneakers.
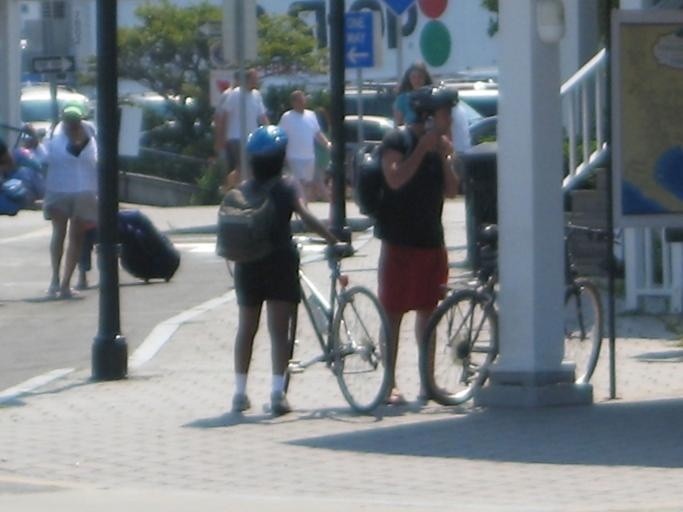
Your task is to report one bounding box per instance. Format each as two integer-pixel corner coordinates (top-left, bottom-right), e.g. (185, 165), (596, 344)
(229, 391), (252, 413)
(269, 395), (292, 415)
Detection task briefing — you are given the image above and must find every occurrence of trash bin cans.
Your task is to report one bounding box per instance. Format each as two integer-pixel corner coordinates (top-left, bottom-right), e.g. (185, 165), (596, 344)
(464, 143), (497, 268)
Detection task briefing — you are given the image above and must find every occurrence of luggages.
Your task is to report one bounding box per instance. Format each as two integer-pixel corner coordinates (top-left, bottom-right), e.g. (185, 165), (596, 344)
(99, 208), (181, 282)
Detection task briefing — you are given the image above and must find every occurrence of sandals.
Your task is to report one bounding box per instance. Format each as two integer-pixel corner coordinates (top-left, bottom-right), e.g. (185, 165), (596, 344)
(59, 286), (73, 299)
(48, 284), (58, 298)
(419, 388), (453, 401)
(382, 387), (406, 405)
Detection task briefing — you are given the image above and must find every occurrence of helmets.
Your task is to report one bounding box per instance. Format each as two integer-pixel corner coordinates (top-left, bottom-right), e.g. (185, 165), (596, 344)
(410, 81), (458, 120)
(247, 125), (289, 161)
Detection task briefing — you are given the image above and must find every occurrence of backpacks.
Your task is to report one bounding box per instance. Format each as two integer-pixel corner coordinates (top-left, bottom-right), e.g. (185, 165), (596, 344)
(214, 189), (285, 262)
(352, 144), (388, 221)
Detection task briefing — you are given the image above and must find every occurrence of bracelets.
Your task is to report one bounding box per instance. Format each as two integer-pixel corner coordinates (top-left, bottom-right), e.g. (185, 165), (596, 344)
(404, 148), (422, 176)
(439, 150), (454, 161)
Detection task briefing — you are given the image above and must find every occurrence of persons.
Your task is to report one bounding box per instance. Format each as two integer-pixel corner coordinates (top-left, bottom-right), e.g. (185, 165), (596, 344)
(391, 61), (433, 126)
(374, 85), (460, 405)
(216, 67), (270, 192)
(22, 107), (95, 301)
(50, 101), (97, 289)
(274, 90), (330, 205)
(232, 123), (336, 417)
(218, 70), (243, 110)
(0, 134), (14, 176)
